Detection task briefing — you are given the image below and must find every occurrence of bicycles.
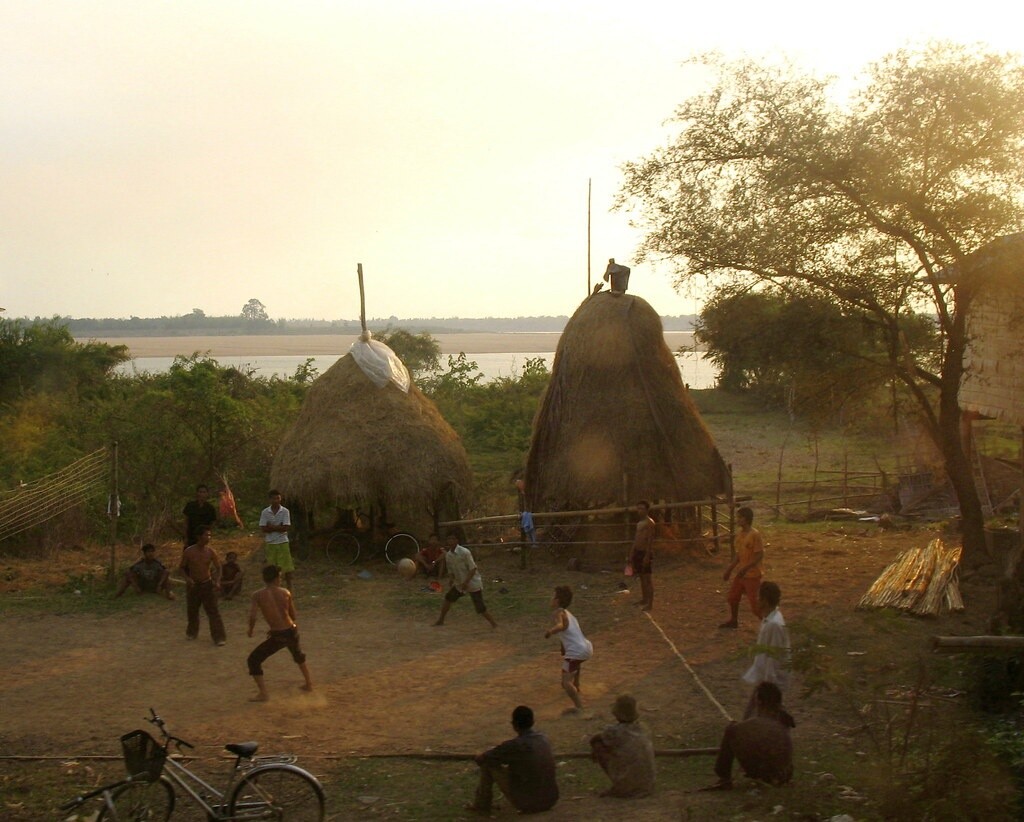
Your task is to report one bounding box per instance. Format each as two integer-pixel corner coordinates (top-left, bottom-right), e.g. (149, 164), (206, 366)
(325, 507), (421, 569)
(97, 708), (326, 822)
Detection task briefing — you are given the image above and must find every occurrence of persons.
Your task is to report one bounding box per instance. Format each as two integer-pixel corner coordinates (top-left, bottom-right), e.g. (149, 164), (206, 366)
(718, 507), (765, 627)
(545, 586), (594, 714)
(741, 581), (793, 720)
(247, 565), (312, 703)
(627, 501), (656, 610)
(462, 705), (559, 813)
(589, 695), (658, 798)
(258, 489), (294, 594)
(713, 681), (796, 788)
(182, 524), (226, 646)
(179, 484), (216, 568)
(430, 534), (498, 633)
(415, 535), (448, 580)
(220, 552), (243, 600)
(116, 543), (176, 600)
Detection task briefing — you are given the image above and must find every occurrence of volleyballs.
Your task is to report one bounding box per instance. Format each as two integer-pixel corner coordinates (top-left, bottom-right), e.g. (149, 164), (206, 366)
(399, 558), (416, 576)
